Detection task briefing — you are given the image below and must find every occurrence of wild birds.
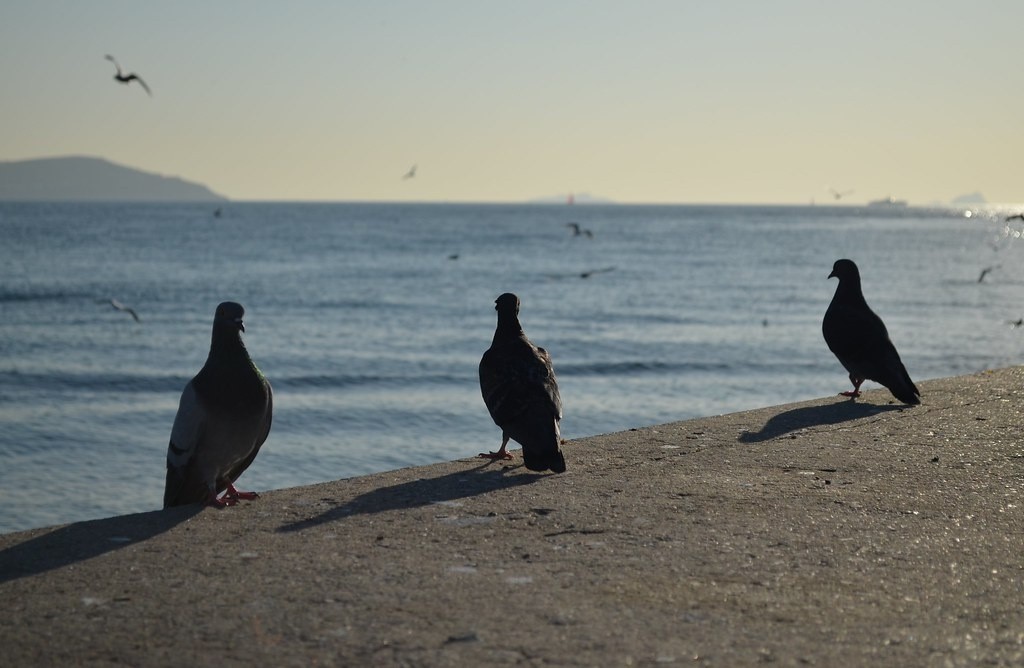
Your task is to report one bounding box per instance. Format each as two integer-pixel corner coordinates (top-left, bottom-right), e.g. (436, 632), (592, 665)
(93, 298), (139, 326)
(161, 302), (274, 529)
(476, 291), (567, 476)
(553, 265), (621, 280)
(823, 258), (924, 411)
(105, 53), (153, 101)
(566, 222), (593, 240)
